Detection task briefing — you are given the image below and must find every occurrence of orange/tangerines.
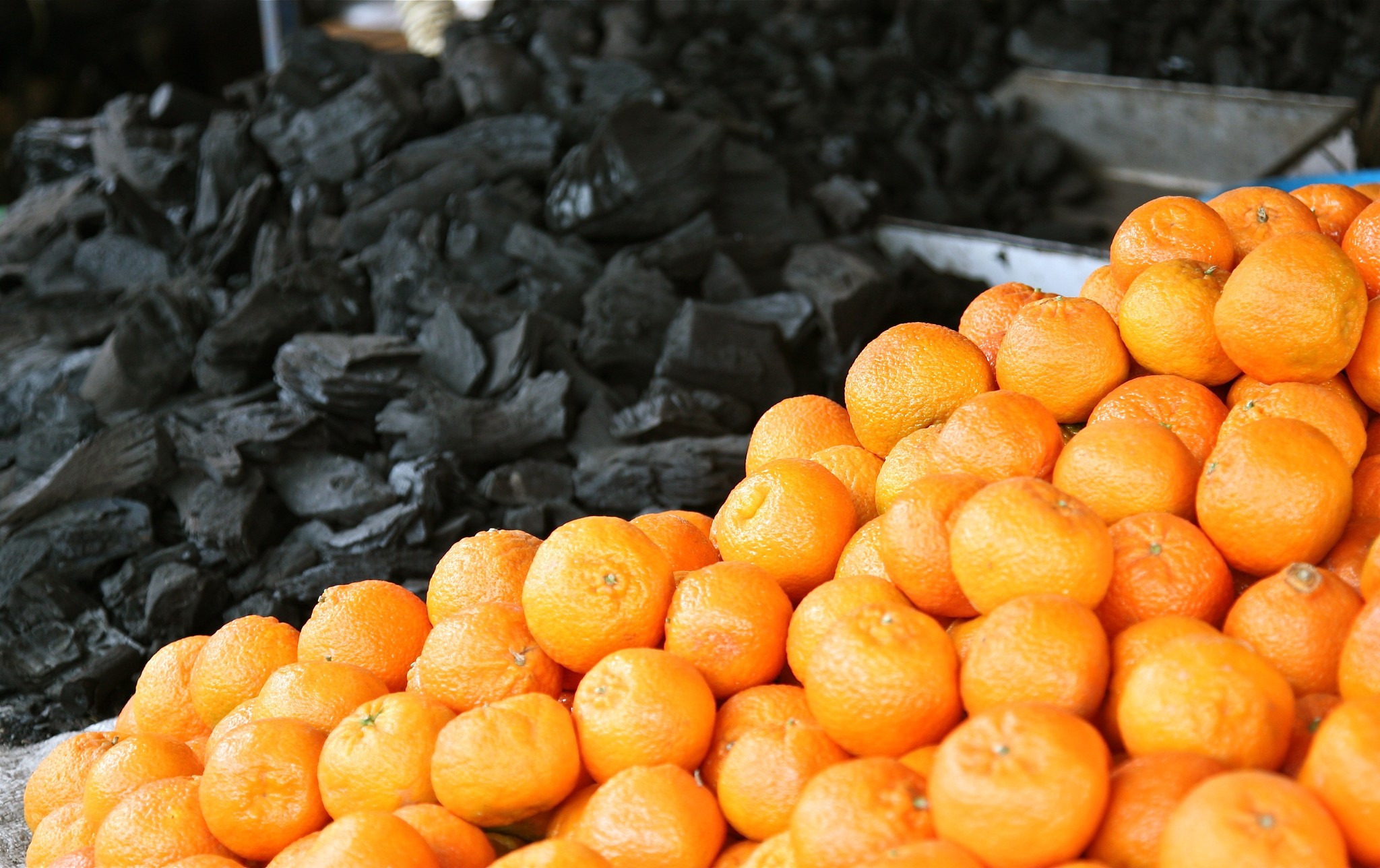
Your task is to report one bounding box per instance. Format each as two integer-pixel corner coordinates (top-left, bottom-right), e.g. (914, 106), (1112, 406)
(14, 103), (1377, 868)
